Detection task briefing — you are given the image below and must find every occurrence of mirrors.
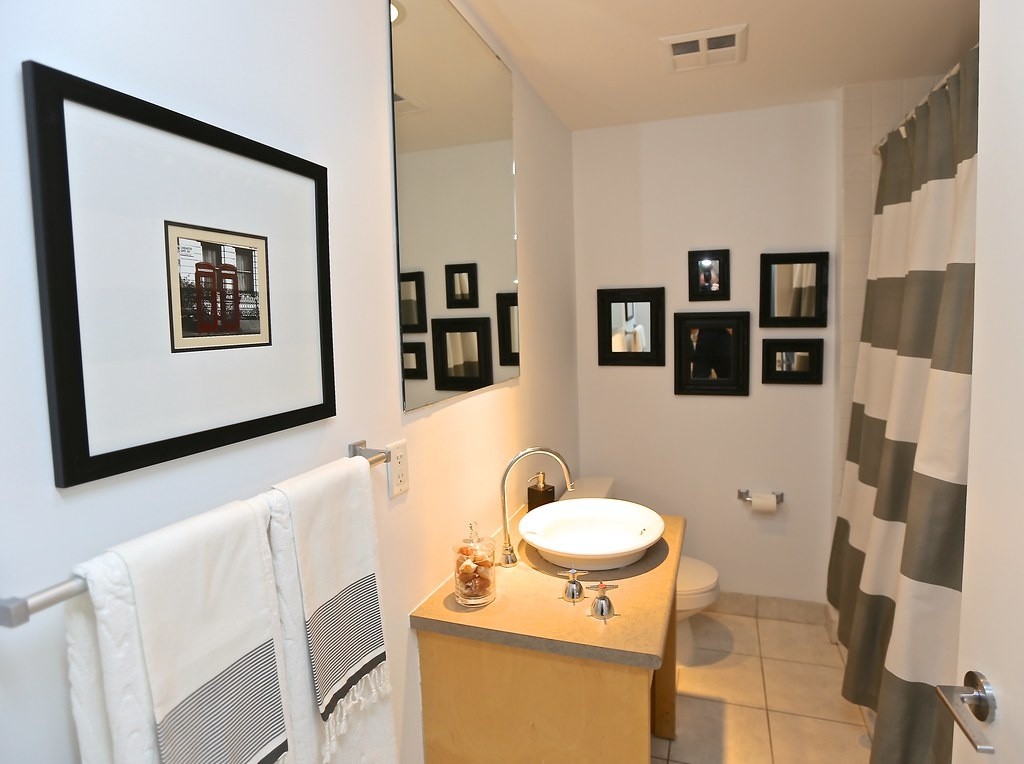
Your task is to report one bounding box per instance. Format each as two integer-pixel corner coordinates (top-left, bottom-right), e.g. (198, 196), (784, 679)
(388, 0), (521, 410)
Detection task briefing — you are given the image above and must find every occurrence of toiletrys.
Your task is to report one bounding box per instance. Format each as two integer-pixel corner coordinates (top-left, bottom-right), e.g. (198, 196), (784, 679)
(527, 472), (557, 514)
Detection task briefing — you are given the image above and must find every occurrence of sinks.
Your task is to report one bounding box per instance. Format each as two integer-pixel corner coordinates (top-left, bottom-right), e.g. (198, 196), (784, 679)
(518, 495), (666, 574)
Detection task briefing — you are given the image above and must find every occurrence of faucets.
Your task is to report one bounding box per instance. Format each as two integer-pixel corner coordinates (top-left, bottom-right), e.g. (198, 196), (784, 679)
(499, 445), (576, 569)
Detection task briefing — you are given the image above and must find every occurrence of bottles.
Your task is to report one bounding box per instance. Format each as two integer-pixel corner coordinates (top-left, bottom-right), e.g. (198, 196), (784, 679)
(454, 522), (497, 606)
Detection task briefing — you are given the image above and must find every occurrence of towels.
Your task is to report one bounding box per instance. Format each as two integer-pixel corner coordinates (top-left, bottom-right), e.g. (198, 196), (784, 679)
(63, 501), (294, 764)
(262, 454), (398, 764)
(631, 324), (647, 353)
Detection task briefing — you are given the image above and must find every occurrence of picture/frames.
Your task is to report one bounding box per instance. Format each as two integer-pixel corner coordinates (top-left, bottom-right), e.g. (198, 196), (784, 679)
(688, 249), (730, 301)
(403, 342), (427, 379)
(445, 263), (478, 308)
(597, 287), (665, 367)
(431, 318), (493, 391)
(496, 293), (519, 366)
(759, 252), (829, 328)
(400, 272), (427, 333)
(674, 311), (750, 396)
(22, 61), (336, 488)
(762, 339), (823, 384)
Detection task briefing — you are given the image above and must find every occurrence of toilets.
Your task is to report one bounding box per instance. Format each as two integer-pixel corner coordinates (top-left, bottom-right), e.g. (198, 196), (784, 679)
(556, 476), (722, 669)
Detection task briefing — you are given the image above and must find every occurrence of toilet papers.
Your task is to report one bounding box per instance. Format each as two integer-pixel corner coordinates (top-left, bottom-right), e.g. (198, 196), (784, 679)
(748, 493), (778, 514)
